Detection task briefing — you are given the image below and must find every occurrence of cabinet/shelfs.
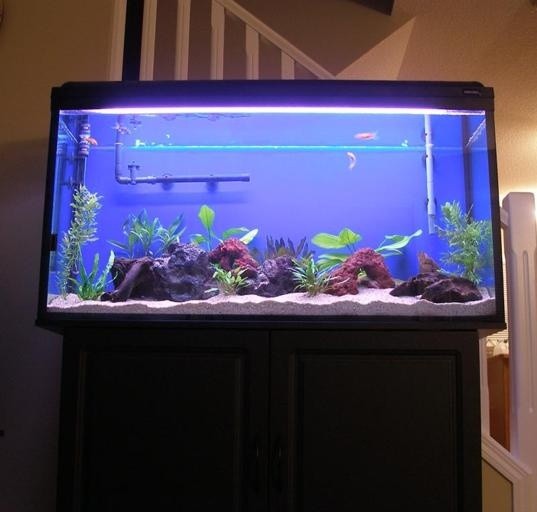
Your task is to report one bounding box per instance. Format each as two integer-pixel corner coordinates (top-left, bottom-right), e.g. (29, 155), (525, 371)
(55, 326), (483, 512)
